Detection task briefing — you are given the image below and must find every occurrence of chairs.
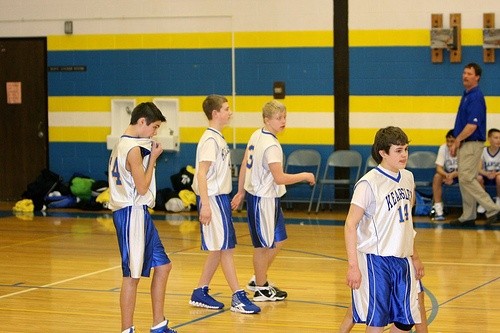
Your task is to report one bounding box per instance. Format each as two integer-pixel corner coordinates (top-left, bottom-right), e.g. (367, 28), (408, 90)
(364, 154), (378, 174)
(284, 149), (322, 213)
(407, 151), (438, 216)
(231, 148), (246, 212)
(316, 150), (361, 214)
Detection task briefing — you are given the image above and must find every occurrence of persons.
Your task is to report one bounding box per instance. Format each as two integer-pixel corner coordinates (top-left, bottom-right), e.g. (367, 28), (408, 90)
(432, 129), (465, 221)
(337, 125), (430, 332)
(229, 100), (317, 301)
(106, 102), (179, 333)
(450, 63), (500, 224)
(188, 94), (260, 314)
(474, 127), (500, 217)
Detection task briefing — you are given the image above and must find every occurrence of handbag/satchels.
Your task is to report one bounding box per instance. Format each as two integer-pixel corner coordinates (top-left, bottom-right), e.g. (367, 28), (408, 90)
(152, 163), (197, 214)
(13, 167), (109, 213)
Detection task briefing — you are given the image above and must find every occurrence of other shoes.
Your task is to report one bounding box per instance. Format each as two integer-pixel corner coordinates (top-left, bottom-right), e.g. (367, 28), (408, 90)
(483, 211), (500, 226)
(150, 320), (178, 333)
(477, 204), (485, 213)
(449, 219), (475, 227)
(432, 201), (447, 220)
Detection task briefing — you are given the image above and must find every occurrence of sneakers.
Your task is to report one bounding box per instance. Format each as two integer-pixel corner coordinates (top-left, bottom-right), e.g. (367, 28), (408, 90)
(253, 281), (288, 302)
(189, 285), (225, 310)
(247, 275), (272, 292)
(230, 289), (261, 314)
